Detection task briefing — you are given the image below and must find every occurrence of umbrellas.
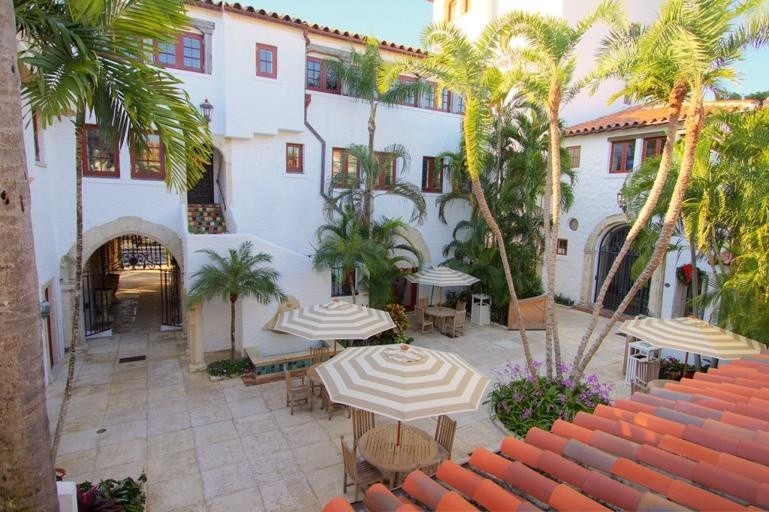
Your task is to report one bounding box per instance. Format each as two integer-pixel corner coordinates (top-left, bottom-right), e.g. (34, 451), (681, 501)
(313, 344), (492, 445)
(618, 313), (767, 378)
(403, 266), (480, 310)
(262, 297), (397, 354)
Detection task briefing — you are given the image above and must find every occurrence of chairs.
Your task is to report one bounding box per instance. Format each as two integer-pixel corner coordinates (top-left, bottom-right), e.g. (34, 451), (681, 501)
(414, 297), (467, 338)
(629, 360), (656, 394)
(284, 347), (352, 421)
(340, 407), (457, 504)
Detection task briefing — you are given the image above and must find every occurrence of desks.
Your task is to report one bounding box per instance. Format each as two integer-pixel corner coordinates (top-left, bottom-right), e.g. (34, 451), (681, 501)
(647, 379), (679, 392)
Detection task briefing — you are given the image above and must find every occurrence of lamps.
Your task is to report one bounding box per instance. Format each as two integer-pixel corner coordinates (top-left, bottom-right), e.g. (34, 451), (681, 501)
(199, 98), (214, 124)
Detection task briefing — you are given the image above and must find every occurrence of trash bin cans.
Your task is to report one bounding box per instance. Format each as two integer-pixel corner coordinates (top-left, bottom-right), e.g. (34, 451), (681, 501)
(625, 341), (663, 390)
(471, 296), (491, 326)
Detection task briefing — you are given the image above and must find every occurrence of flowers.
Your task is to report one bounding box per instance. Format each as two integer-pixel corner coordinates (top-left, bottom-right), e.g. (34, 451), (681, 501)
(659, 356), (695, 381)
(482, 357), (617, 443)
(55, 466), (147, 512)
